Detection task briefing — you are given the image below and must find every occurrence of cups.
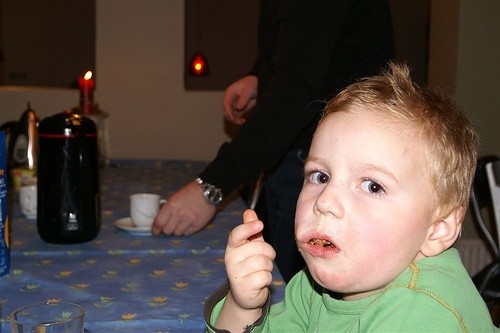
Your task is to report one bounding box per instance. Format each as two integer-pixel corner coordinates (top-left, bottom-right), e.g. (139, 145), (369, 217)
(8, 300), (85, 333)
(19, 186), (38, 221)
(130, 194), (167, 227)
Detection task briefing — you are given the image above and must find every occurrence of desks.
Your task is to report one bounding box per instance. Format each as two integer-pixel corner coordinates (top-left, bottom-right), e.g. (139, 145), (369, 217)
(1, 158), (286, 333)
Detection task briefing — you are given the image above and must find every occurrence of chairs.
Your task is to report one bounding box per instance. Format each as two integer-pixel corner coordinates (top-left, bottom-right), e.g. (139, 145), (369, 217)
(462, 154), (500, 312)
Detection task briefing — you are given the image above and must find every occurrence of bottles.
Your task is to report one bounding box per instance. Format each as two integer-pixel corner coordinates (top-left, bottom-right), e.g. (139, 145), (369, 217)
(35, 110), (102, 243)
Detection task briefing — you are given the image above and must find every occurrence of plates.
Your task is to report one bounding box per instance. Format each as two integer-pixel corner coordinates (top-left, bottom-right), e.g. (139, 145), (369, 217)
(114, 217), (153, 237)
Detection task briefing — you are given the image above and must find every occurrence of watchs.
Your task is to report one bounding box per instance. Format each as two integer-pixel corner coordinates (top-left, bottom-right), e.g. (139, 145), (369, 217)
(193, 178), (222, 205)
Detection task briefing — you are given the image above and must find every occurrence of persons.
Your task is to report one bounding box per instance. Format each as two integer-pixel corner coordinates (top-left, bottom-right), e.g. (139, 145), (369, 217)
(152, 0), (395, 280)
(200, 63), (500, 333)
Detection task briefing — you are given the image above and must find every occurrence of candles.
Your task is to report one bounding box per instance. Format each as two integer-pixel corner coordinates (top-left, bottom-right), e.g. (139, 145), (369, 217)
(77, 71), (97, 115)
